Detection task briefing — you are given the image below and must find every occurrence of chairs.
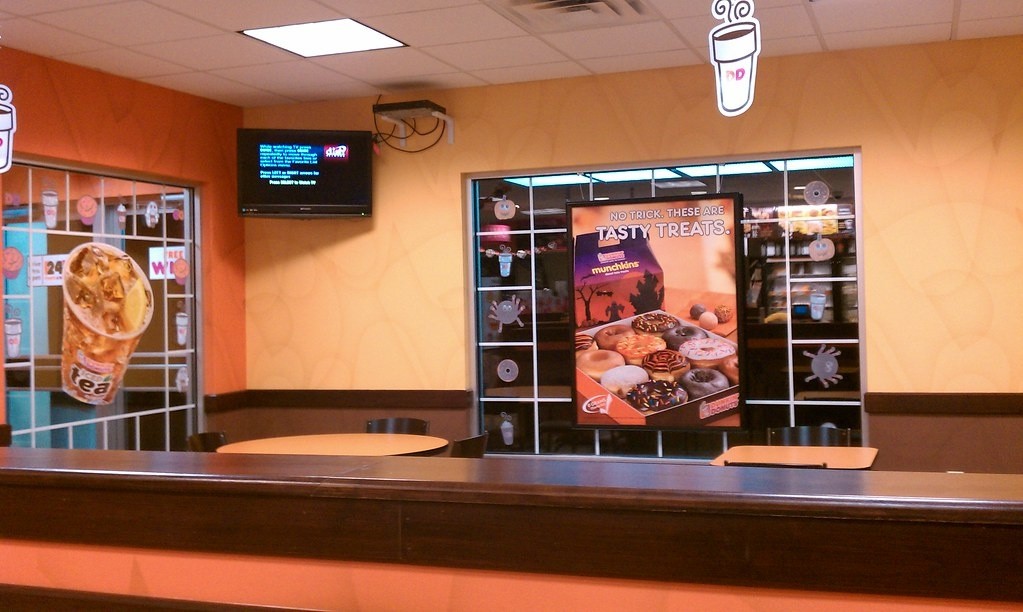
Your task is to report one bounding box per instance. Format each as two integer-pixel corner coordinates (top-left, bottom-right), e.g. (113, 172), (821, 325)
(186, 430), (226, 453)
(766, 427), (852, 446)
(723, 460), (828, 471)
(450, 431), (490, 459)
(365, 418), (431, 437)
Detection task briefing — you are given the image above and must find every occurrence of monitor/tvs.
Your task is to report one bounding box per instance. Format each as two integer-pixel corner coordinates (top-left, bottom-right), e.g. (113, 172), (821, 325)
(236, 128), (374, 217)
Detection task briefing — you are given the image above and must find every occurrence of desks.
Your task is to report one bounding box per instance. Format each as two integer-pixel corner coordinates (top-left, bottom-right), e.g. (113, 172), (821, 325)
(710, 445), (880, 470)
(216, 432), (448, 457)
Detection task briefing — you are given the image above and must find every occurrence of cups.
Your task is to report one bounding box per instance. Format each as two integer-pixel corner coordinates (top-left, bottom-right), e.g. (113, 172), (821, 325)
(59, 241), (154, 407)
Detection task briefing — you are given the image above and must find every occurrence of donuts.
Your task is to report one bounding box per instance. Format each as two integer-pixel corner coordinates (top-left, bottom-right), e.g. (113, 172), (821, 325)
(574, 311), (739, 413)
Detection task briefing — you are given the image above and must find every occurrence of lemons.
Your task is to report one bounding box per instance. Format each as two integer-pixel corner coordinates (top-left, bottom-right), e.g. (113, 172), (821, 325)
(120, 279), (147, 332)
